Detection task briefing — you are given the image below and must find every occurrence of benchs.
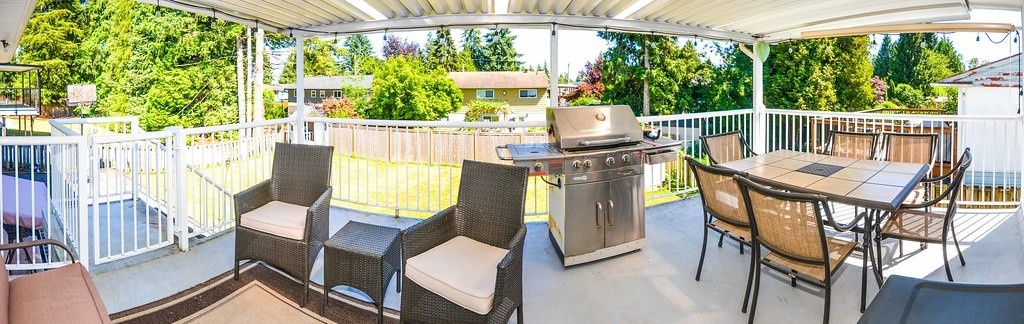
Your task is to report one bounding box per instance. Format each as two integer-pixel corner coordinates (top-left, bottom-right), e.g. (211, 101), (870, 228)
(0, 240), (111, 324)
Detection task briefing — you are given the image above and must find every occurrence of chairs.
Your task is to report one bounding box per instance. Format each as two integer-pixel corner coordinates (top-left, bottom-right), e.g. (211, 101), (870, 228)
(686, 156), (798, 313)
(734, 174), (870, 324)
(398, 160), (530, 324)
(864, 132), (940, 257)
(233, 142), (334, 307)
(876, 147), (972, 283)
(698, 131), (775, 221)
(815, 130), (882, 243)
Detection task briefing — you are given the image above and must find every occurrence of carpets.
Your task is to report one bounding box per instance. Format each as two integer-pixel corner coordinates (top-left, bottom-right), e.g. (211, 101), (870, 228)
(107, 258), (401, 324)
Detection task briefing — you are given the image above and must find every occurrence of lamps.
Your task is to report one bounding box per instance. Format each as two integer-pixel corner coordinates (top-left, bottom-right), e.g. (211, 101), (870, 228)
(1013, 34), (1017, 47)
(605, 28), (609, 40)
(383, 29), (388, 40)
(694, 35), (697, 46)
(729, 39), (733, 49)
(156, 0), (161, 18)
(211, 9), (217, 28)
(495, 24), (498, 35)
(439, 26), (445, 38)
(975, 33), (980, 47)
(1, 40), (13, 53)
(827, 37), (830, 52)
(289, 29), (293, 38)
(551, 24), (555, 35)
(872, 34), (876, 49)
(334, 32), (338, 43)
(789, 39), (792, 53)
(921, 32), (925, 49)
(253, 20), (258, 38)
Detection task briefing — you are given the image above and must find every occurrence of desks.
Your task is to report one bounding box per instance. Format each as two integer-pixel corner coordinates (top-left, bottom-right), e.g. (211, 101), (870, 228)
(711, 148), (931, 291)
(322, 220), (401, 324)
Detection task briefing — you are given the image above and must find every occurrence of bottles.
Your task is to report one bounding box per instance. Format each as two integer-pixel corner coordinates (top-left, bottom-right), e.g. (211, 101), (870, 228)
(637, 110), (657, 133)
